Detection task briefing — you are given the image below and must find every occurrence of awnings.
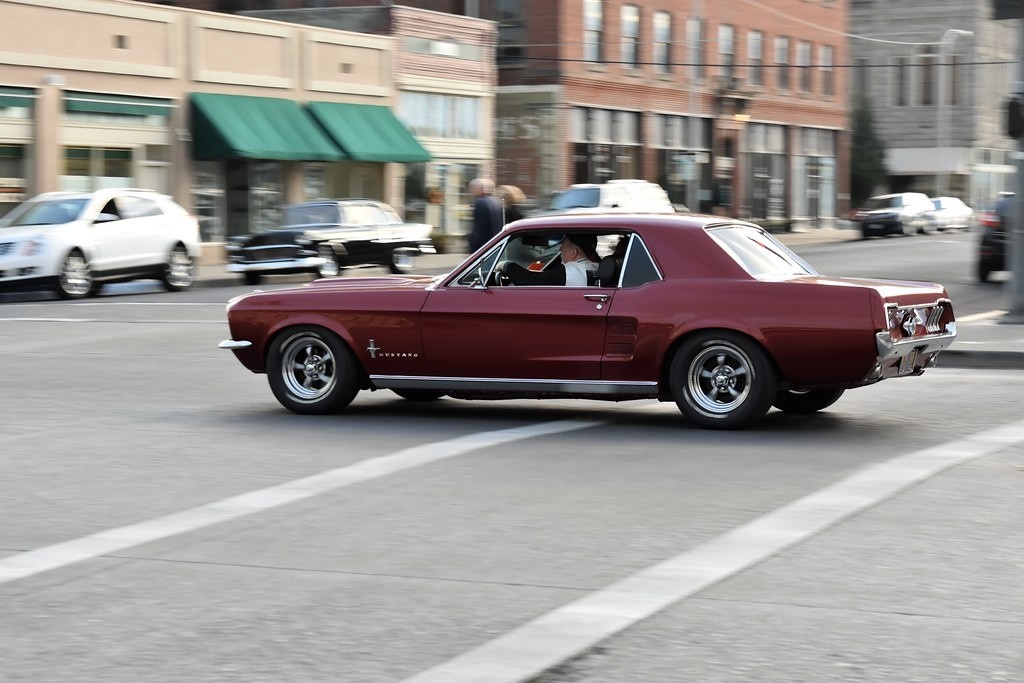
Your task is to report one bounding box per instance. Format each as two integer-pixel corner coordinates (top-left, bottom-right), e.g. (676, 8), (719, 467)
(187, 91), (430, 162)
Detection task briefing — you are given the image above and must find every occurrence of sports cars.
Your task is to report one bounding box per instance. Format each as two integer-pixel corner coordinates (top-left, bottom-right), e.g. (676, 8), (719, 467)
(213, 200), (960, 431)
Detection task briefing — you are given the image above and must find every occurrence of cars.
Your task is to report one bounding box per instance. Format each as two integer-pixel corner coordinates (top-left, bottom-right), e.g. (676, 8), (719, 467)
(971, 192), (1016, 281)
(932, 196), (978, 233)
(220, 195), (436, 285)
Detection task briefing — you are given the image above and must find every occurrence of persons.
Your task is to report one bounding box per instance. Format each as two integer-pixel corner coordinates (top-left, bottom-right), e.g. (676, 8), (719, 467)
(467, 178), (528, 251)
(494, 230), (600, 287)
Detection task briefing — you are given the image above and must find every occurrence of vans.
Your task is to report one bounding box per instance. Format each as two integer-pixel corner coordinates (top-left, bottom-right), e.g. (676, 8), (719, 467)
(547, 175), (676, 259)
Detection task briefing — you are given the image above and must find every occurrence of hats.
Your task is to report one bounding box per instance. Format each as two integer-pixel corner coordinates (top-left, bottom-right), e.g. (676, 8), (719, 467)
(566, 233), (602, 262)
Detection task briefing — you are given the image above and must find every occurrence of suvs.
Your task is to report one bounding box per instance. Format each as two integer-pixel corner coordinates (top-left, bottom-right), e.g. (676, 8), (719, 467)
(857, 192), (939, 240)
(0, 187), (204, 300)
